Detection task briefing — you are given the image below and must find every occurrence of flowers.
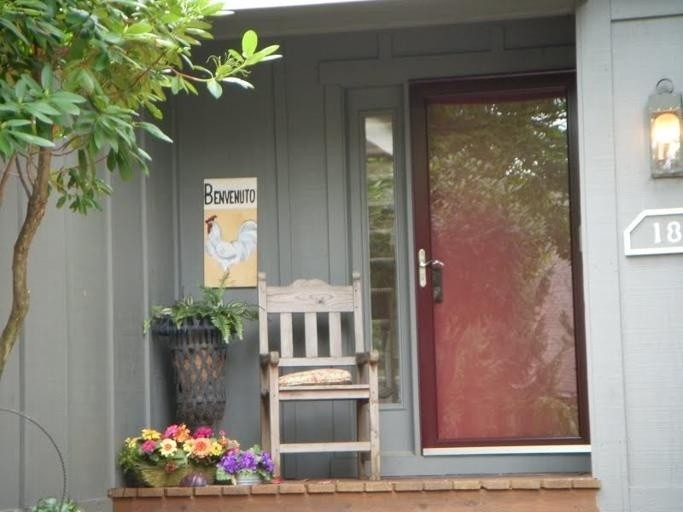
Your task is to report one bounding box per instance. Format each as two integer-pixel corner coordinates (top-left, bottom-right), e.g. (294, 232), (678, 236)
(117, 424), (277, 473)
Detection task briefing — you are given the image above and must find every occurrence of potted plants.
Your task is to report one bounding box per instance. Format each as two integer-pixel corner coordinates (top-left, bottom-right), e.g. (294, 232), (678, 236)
(143, 270), (259, 436)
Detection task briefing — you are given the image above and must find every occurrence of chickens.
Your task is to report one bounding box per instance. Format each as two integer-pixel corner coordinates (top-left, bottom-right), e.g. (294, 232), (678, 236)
(205, 215), (258, 286)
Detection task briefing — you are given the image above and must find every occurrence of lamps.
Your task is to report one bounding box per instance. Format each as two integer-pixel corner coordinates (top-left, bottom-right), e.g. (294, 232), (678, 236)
(645, 78), (682, 180)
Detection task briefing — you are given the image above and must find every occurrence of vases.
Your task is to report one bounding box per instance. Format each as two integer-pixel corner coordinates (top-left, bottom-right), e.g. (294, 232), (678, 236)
(133, 461), (267, 487)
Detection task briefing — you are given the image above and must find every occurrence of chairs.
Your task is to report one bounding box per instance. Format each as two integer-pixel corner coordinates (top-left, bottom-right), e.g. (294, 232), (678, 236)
(257, 271), (381, 482)
